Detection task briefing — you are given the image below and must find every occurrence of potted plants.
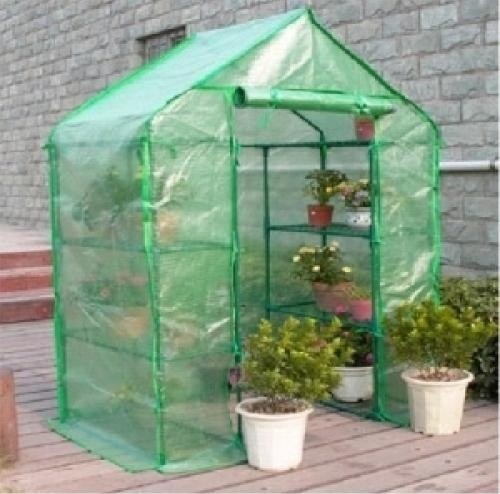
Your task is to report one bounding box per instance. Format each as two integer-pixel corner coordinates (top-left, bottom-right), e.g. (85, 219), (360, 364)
(380, 299), (498, 439)
(225, 312), (355, 473)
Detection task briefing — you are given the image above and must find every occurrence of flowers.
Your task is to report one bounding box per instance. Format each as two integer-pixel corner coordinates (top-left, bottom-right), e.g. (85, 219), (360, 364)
(275, 165), (375, 406)
(69, 158), (199, 432)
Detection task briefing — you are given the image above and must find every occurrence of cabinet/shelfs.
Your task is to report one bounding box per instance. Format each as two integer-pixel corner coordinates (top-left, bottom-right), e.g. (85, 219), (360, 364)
(54, 137), (239, 464)
(263, 138), (377, 421)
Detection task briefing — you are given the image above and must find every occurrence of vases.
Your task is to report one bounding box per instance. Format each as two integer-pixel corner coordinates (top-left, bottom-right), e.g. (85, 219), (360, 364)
(353, 114), (374, 143)
